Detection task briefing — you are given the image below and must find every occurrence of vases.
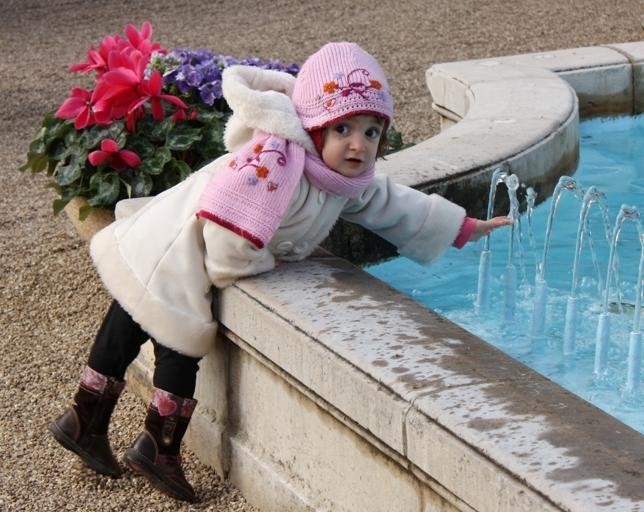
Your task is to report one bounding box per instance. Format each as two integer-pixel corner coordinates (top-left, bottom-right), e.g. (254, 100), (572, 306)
(63, 195), (117, 239)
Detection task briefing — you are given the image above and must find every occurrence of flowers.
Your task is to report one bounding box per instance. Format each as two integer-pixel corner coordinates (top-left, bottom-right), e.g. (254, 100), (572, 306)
(19, 28), (305, 222)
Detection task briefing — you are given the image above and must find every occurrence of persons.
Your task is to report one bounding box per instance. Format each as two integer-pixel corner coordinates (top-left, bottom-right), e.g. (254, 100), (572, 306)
(48, 41), (515, 500)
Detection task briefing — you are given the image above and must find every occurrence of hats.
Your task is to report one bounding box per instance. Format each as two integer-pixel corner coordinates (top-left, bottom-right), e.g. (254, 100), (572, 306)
(290, 39), (394, 132)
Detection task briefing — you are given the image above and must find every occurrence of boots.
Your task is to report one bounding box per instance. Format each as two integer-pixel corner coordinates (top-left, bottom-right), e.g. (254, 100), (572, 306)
(48, 365), (127, 482)
(121, 386), (198, 504)
